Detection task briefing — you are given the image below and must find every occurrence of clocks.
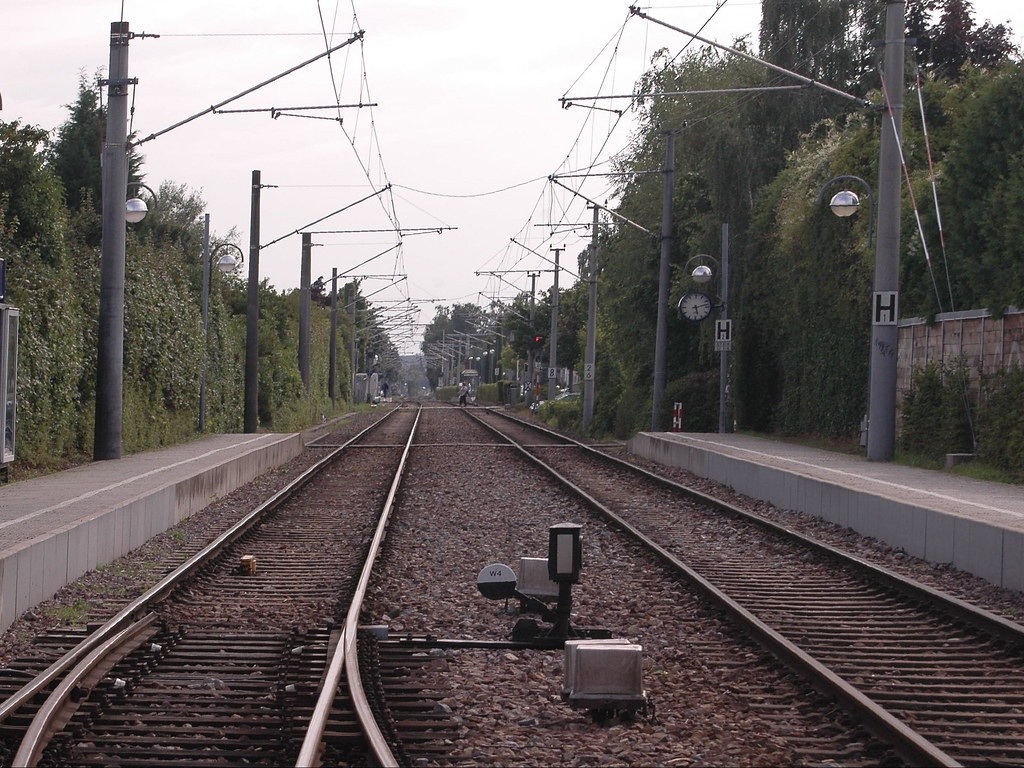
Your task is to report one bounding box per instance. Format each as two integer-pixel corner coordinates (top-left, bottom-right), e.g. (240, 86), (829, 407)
(677, 292), (723, 322)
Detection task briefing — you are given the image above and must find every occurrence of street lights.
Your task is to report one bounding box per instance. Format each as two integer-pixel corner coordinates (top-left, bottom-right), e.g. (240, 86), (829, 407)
(93, 182), (158, 461)
(685, 222), (728, 435)
(820, 176), (900, 463)
(199, 213), (244, 433)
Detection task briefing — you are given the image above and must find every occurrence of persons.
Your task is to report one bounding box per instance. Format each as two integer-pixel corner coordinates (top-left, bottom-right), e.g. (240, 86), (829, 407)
(457, 383), (469, 407)
(381, 381), (388, 398)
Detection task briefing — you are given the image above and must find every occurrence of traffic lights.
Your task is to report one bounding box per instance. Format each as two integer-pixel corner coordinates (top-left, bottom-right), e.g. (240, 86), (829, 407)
(529, 333), (545, 347)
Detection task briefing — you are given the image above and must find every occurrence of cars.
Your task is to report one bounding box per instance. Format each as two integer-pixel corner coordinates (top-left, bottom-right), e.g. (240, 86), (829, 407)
(519, 382), (581, 415)
